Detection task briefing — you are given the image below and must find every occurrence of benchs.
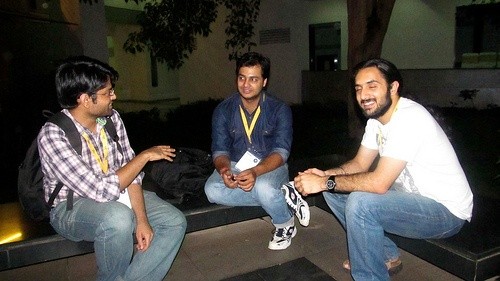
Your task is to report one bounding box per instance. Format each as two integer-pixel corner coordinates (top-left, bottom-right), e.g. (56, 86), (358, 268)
(0, 137), (500, 280)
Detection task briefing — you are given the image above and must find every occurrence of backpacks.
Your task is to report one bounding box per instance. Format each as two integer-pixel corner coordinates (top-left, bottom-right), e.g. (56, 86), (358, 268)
(16, 110), (119, 220)
(149, 145), (218, 204)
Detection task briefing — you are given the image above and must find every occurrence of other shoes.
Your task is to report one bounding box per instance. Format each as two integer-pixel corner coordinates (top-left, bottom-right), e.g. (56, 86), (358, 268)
(342, 257), (403, 274)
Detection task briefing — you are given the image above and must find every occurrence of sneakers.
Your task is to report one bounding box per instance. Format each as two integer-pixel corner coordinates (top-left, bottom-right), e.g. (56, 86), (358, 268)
(281, 180), (310, 227)
(268, 217), (298, 250)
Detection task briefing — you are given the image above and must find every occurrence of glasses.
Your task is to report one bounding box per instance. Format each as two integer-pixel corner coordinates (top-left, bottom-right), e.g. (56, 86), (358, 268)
(89, 89), (115, 96)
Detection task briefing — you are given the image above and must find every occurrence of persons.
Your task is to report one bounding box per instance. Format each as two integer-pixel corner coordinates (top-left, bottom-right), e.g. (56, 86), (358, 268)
(294, 57), (474, 281)
(37, 56), (189, 280)
(203, 52), (311, 250)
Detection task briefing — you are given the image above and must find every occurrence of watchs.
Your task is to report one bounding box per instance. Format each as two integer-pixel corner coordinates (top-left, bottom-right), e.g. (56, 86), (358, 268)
(326, 175), (336, 193)
